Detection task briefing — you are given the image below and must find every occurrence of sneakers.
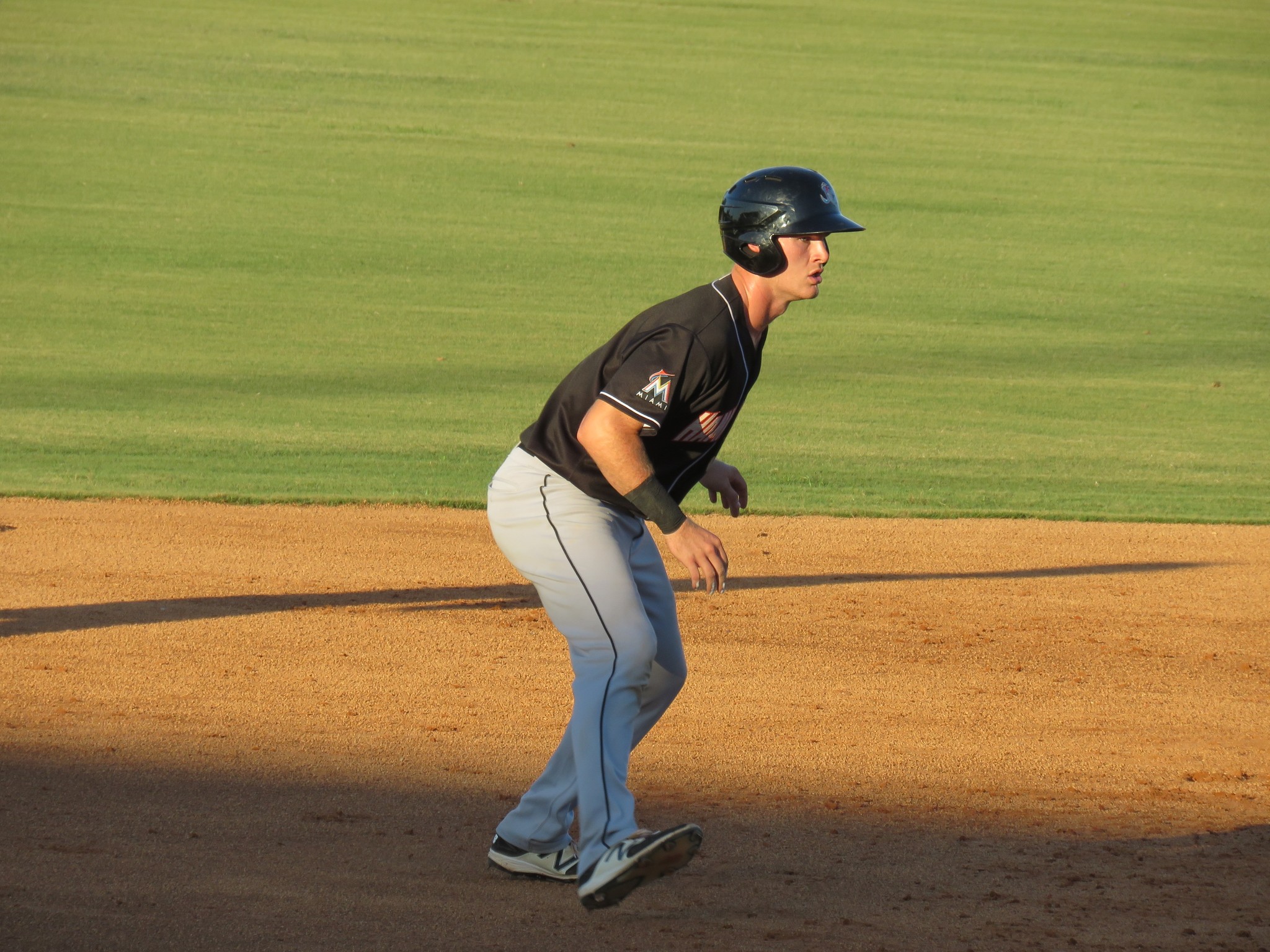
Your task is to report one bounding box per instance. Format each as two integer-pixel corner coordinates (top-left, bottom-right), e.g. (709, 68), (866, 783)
(487, 833), (579, 879)
(577, 823), (704, 911)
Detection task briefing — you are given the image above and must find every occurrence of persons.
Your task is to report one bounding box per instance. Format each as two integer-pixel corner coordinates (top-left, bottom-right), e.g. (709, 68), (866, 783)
(486, 168), (865, 909)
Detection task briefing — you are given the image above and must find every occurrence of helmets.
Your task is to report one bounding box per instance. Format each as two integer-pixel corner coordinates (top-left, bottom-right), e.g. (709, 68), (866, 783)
(719, 167), (866, 279)
